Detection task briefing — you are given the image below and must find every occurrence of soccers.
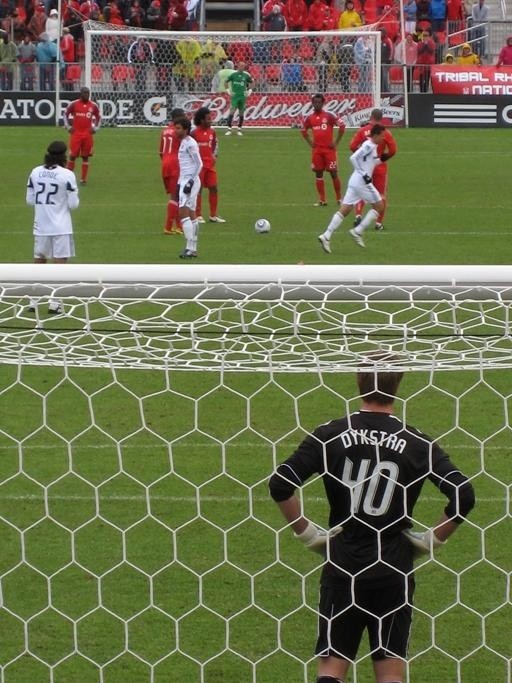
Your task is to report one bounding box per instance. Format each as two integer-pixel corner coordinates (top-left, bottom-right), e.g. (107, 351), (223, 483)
(255, 218), (271, 234)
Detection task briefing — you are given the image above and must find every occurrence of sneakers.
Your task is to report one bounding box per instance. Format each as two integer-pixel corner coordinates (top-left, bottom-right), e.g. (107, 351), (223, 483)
(48, 303), (66, 314)
(353, 215), (362, 227)
(225, 127), (232, 136)
(180, 249), (192, 258)
(175, 227), (183, 234)
(236, 127), (243, 135)
(164, 228), (176, 234)
(192, 251), (197, 256)
(375, 222), (384, 230)
(28, 302), (38, 312)
(317, 234), (332, 254)
(79, 180), (87, 185)
(314, 201), (327, 207)
(209, 216), (226, 223)
(197, 216), (206, 223)
(348, 229), (365, 248)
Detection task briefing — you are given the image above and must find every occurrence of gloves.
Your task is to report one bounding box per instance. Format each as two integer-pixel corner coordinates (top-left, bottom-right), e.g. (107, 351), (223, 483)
(294, 522), (343, 556)
(247, 89), (252, 97)
(183, 179), (194, 194)
(401, 527), (447, 560)
(225, 88), (231, 96)
(363, 174), (373, 184)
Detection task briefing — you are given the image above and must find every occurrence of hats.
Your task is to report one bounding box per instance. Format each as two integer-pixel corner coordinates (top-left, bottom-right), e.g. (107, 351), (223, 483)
(49, 9), (58, 16)
(62, 27), (70, 34)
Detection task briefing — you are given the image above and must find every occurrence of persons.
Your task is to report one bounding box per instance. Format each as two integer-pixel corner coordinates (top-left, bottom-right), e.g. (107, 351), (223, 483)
(401, 35), (417, 93)
(418, 0), (431, 20)
(59, 26), (75, 65)
(317, 124), (385, 254)
(81, 0), (101, 20)
(30, 3), (49, 41)
(292, 94), (345, 214)
(173, 118), (203, 259)
(1, 30), (20, 91)
(174, 35), (201, 93)
(159, 108), (185, 234)
(63, 87), (102, 186)
(168, 1), (186, 30)
(354, 33), (373, 93)
(25, 141), (79, 315)
(262, 2), (286, 31)
(45, 9), (61, 44)
(125, 0), (145, 27)
(105, 1), (122, 24)
(403, 0), (416, 34)
(61, 0), (80, 16)
(470, 0), (487, 58)
(188, 108), (226, 223)
(279, 52), (307, 90)
(147, 1), (165, 28)
(211, 59), (235, 92)
(337, 38), (354, 92)
(184, 0), (199, 30)
(497, 33), (511, 68)
(36, 33), (65, 90)
(442, 54), (455, 66)
(228, 37), (357, 94)
(64, 12), (84, 41)
(315, 35), (336, 92)
(308, 2), (329, 30)
(200, 38), (228, 91)
(349, 109), (397, 230)
(447, 1), (465, 33)
(17, 36), (38, 92)
(128, 36), (154, 92)
(109, 39), (129, 93)
(224, 62), (254, 136)
(419, 31), (437, 92)
(364, 0), (395, 25)
(155, 38), (182, 92)
(457, 42), (480, 65)
(9, 7), (26, 39)
(271, 353), (474, 683)
(338, 0), (360, 28)
(288, 0), (307, 31)
(378, 28), (393, 94)
(433, 2), (445, 30)
